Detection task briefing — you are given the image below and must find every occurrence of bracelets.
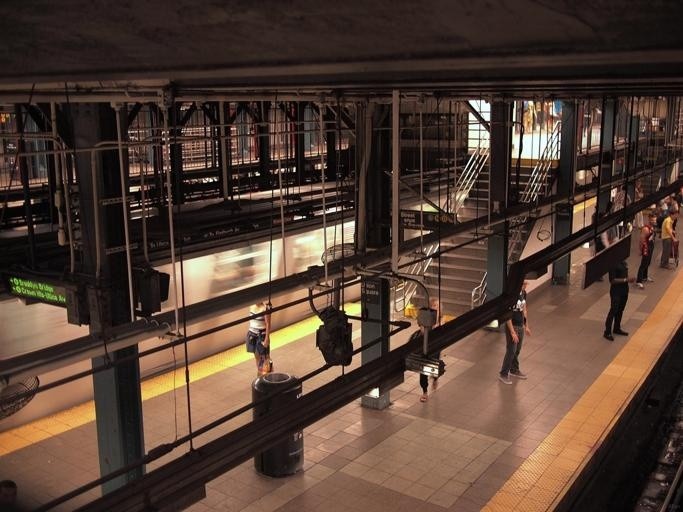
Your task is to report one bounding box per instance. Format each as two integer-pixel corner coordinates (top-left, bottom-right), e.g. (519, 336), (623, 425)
(513, 334), (516, 342)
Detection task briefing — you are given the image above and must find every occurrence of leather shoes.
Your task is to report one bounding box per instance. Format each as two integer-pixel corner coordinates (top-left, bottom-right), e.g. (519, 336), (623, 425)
(603, 330), (615, 342)
(612, 327), (630, 336)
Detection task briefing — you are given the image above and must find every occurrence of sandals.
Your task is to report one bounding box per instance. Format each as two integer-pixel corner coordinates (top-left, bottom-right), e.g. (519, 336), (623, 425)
(431, 379), (439, 391)
(419, 392), (429, 403)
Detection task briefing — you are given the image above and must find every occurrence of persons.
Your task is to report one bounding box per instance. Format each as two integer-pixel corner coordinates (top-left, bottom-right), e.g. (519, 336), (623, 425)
(429, 297), (443, 329)
(590, 179), (683, 341)
(497, 280), (532, 385)
(410, 325), (445, 402)
(246, 300), (271, 376)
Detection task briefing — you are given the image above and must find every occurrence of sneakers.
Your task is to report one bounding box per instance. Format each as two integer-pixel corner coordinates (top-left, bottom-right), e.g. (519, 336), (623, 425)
(636, 282), (644, 289)
(660, 263), (675, 271)
(509, 369), (528, 381)
(497, 372), (513, 385)
(641, 277), (654, 282)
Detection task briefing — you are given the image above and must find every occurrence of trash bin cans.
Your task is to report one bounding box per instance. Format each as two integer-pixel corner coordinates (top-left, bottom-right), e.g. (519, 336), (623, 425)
(251, 373), (303, 478)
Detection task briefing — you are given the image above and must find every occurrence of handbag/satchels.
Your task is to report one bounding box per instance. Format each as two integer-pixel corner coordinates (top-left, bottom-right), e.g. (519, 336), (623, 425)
(256, 345), (274, 378)
(249, 335), (256, 346)
